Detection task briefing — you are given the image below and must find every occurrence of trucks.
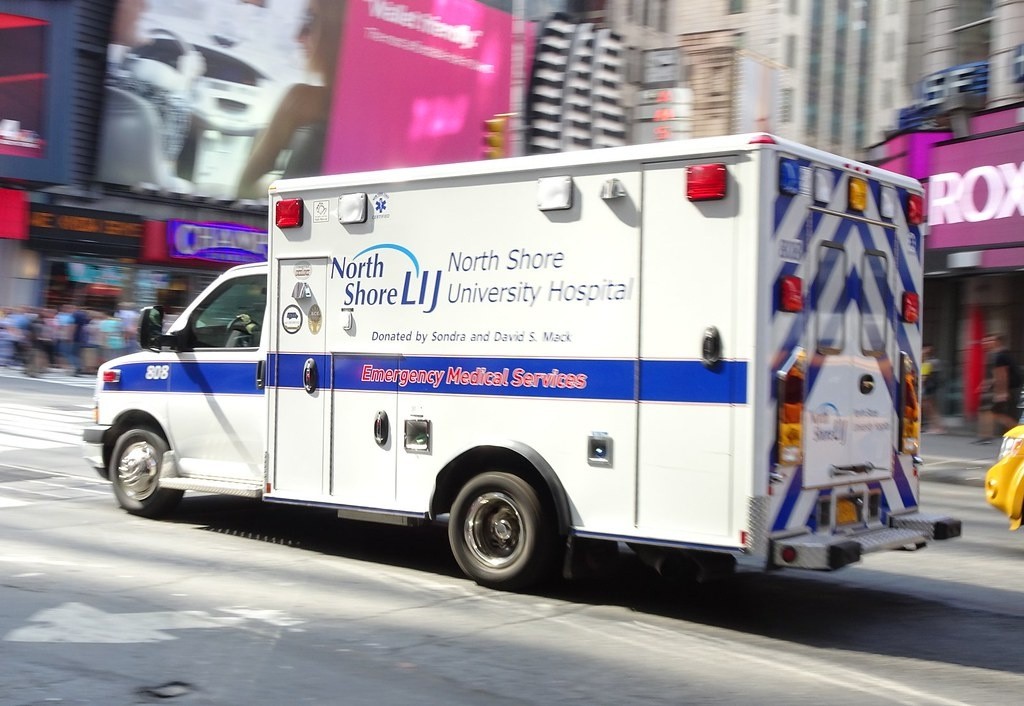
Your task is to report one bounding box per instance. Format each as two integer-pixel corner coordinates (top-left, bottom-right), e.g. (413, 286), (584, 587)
(80, 132), (962, 586)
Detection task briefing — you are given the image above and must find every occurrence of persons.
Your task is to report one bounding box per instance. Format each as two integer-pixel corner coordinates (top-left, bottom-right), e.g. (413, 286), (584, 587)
(970, 331), (1024, 444)
(922, 347), (949, 433)
(105, 1), (345, 199)
(0, 302), (141, 380)
